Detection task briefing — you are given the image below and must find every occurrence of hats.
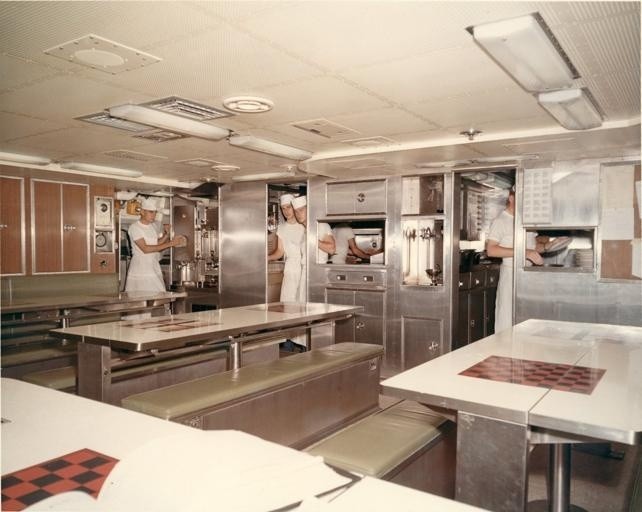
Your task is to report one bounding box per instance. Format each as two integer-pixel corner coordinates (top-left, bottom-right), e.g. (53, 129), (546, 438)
(281, 195), (294, 206)
(293, 195), (307, 209)
(142, 201), (157, 212)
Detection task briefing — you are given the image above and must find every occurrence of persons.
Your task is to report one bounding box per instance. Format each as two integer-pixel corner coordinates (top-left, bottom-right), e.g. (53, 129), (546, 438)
(292, 196), (336, 354)
(269, 195), (305, 352)
(486, 184), (568, 336)
(122, 199), (185, 320)
(327, 219), (381, 265)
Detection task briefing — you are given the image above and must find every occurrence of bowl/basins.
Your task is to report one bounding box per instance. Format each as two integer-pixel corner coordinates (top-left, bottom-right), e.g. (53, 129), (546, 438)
(458, 249), (477, 273)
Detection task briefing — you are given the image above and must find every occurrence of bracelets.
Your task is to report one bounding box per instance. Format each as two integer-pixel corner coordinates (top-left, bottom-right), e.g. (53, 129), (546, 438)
(543, 243), (548, 251)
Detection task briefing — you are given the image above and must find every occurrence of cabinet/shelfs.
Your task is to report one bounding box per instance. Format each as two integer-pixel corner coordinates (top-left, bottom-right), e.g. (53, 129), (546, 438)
(0, 174), (26, 277)
(325, 289), (384, 346)
(29, 178), (90, 276)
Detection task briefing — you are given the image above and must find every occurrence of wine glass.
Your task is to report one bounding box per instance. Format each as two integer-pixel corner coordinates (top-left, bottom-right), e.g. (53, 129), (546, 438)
(426, 268), (440, 285)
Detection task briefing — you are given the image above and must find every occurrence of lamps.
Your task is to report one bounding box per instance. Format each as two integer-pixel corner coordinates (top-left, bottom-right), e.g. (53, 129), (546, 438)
(60, 162), (143, 178)
(473, 15), (573, 93)
(0, 151), (50, 166)
(227, 136), (312, 161)
(108, 104), (231, 140)
(232, 169), (292, 182)
(536, 89), (602, 131)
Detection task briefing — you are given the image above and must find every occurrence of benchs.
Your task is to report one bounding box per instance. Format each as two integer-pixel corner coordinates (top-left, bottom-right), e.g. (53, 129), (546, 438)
(300, 396), (456, 500)
(122, 339), (384, 451)
(20, 337), (287, 409)
(1, 313), (122, 378)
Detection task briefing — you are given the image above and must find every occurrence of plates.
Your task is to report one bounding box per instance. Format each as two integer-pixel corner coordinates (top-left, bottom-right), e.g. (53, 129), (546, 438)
(575, 249), (593, 268)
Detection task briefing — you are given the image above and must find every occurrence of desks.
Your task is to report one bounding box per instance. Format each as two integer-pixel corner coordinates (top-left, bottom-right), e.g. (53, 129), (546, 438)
(377, 317), (642, 512)
(2, 377), (494, 512)
(50, 301), (365, 408)
(1, 289), (188, 346)
